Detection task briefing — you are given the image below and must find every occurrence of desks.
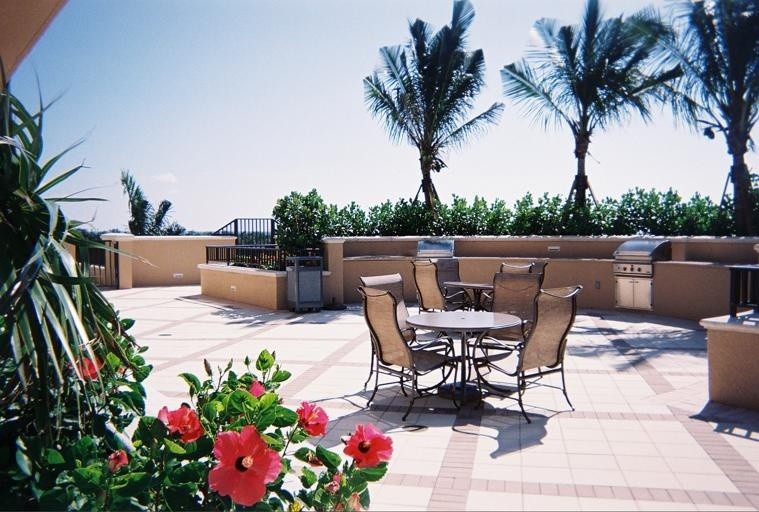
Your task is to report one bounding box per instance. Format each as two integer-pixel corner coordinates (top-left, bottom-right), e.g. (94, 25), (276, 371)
(406, 311), (522, 408)
(443, 281), (494, 338)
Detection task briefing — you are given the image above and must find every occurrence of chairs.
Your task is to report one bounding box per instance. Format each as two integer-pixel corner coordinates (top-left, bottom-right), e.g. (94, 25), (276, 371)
(466, 283), (583, 425)
(467, 273), (543, 387)
(430, 259), (473, 311)
(357, 286), (461, 420)
(358, 272), (456, 397)
(410, 259), (465, 314)
(484, 262), (535, 307)
(531, 261), (548, 288)
